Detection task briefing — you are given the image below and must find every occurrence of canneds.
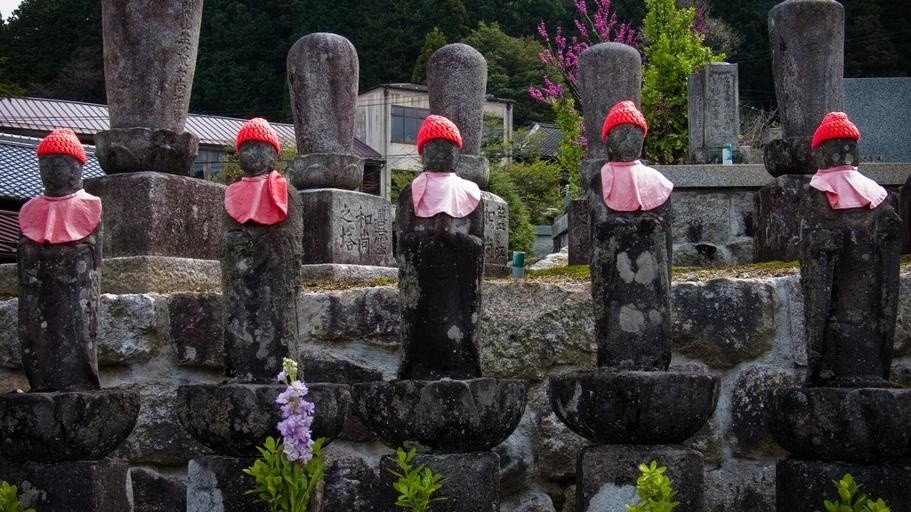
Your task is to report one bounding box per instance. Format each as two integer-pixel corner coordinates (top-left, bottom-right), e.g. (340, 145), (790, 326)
(722, 143), (733, 165)
(512, 251), (525, 278)
